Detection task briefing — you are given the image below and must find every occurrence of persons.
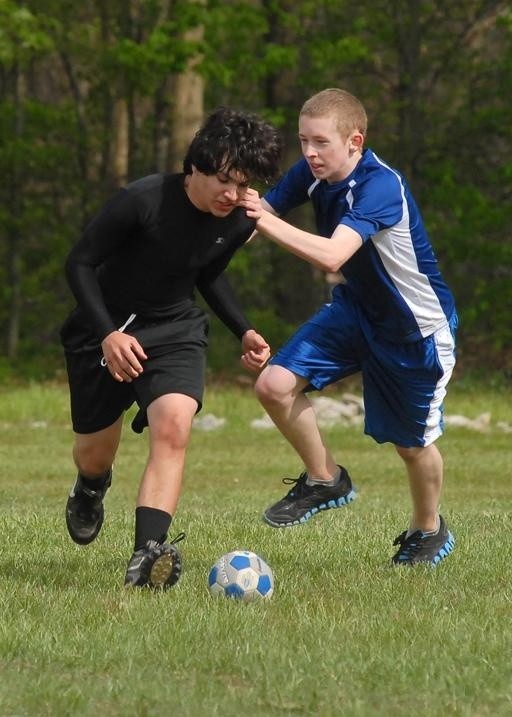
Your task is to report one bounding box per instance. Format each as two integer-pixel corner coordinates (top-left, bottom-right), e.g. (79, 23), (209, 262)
(56, 105), (277, 591)
(231, 84), (463, 575)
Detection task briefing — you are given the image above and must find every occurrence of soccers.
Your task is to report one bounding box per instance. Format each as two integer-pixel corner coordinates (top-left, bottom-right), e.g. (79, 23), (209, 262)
(207, 550), (276, 605)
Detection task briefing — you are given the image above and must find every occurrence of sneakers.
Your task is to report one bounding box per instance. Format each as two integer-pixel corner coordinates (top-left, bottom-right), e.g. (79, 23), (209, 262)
(262, 465), (356, 528)
(391, 514), (455, 570)
(66, 464), (113, 545)
(124, 533), (186, 593)
